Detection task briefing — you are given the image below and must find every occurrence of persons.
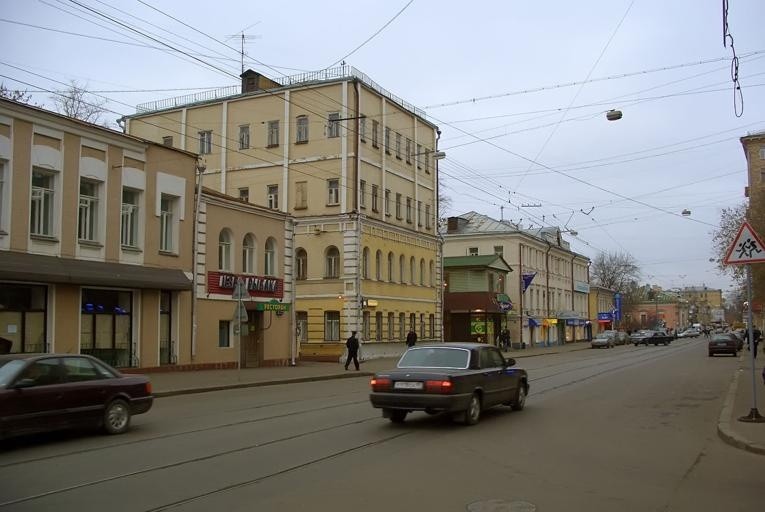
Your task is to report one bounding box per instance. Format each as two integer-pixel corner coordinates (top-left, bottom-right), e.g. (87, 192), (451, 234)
(502, 326), (510, 345)
(406, 327), (417, 348)
(626, 325), (761, 359)
(345, 331), (359, 370)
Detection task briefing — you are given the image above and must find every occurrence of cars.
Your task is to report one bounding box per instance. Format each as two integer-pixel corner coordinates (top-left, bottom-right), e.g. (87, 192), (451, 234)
(1, 353), (153, 440)
(591, 323), (746, 357)
(369, 342), (529, 425)
